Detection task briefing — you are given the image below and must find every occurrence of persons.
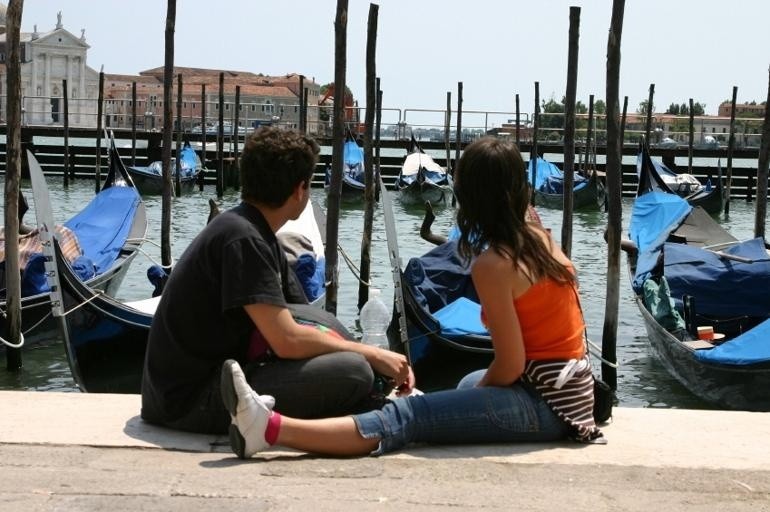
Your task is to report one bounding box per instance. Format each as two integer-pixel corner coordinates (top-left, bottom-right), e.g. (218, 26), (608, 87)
(219, 138), (595, 459)
(140, 125), (416, 434)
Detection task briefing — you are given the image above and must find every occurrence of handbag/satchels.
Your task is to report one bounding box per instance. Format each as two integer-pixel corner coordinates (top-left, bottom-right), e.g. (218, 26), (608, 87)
(592, 379), (612, 423)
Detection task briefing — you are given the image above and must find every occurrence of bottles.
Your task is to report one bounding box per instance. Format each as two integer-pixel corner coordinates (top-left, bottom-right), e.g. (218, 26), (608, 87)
(358, 288), (390, 350)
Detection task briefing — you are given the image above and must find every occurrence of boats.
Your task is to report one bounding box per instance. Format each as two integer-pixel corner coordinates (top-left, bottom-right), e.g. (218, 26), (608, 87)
(395, 129), (454, 208)
(387, 237), (494, 393)
(526, 141), (601, 213)
(627, 134), (770, 409)
(324, 123), (376, 204)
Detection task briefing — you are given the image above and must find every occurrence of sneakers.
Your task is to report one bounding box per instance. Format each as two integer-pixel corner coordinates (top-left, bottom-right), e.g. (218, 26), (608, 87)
(221, 360), (275, 460)
